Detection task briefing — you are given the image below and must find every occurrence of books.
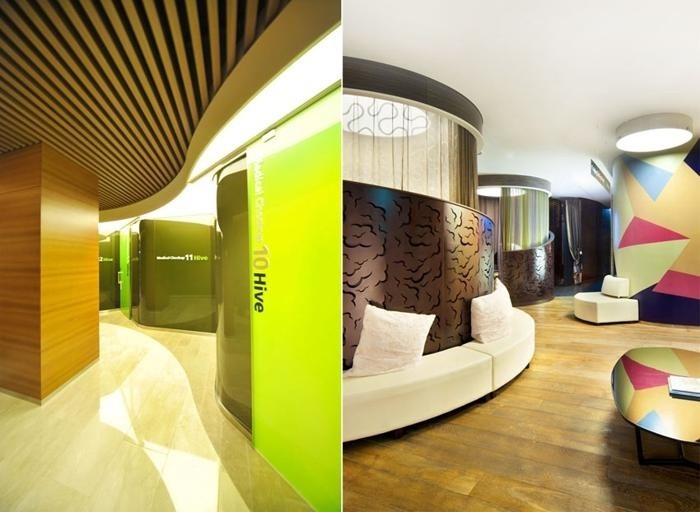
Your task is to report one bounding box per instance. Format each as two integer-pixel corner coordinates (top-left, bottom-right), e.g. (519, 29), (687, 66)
(668, 375), (700, 401)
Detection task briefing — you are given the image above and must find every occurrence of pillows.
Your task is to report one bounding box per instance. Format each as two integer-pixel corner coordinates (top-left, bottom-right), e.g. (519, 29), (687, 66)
(470, 276), (516, 344)
(343, 304), (437, 378)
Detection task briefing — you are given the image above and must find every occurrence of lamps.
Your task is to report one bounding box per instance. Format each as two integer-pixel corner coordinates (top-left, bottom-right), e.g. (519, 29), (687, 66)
(615, 112), (693, 153)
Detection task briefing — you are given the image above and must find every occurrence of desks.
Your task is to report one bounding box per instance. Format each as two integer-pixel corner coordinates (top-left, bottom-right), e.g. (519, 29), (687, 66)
(611, 346), (700, 466)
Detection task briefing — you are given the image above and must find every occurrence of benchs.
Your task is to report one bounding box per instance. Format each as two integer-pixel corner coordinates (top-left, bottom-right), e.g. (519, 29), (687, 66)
(573, 275), (640, 325)
(341, 306), (534, 443)
